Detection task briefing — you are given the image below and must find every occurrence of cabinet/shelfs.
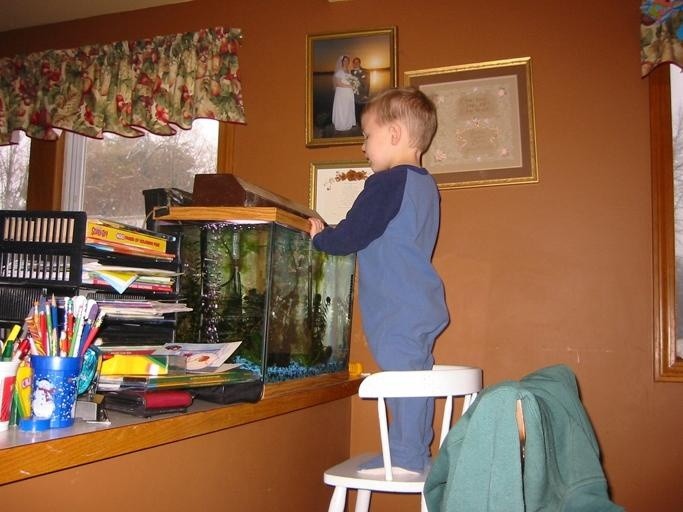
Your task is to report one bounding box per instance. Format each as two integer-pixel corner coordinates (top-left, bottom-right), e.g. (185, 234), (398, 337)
(0, 209), (185, 351)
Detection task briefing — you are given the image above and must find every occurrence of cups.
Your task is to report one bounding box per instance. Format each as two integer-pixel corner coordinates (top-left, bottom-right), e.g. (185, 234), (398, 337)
(0, 357), (22, 431)
(27, 353), (84, 431)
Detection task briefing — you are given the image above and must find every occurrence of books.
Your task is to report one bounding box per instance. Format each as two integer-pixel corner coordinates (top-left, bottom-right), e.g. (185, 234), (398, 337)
(97, 339), (257, 391)
(2, 219), (193, 321)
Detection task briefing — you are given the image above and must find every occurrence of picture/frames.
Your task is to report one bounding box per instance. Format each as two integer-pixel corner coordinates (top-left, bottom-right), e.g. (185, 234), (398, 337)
(648, 60), (682, 384)
(400, 55), (540, 191)
(303, 23), (399, 149)
(306, 160), (375, 230)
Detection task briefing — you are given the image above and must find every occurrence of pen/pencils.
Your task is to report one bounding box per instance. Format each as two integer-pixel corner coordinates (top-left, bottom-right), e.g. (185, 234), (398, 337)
(0, 325), (28, 363)
(23, 291), (106, 358)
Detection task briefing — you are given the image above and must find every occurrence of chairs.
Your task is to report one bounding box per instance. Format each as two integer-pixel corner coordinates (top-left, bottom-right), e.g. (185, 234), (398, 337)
(319, 363), (484, 512)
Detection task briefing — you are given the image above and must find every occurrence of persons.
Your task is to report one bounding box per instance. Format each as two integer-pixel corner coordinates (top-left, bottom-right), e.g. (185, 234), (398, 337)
(350, 57), (369, 124)
(330, 54), (357, 132)
(307, 84), (451, 479)
